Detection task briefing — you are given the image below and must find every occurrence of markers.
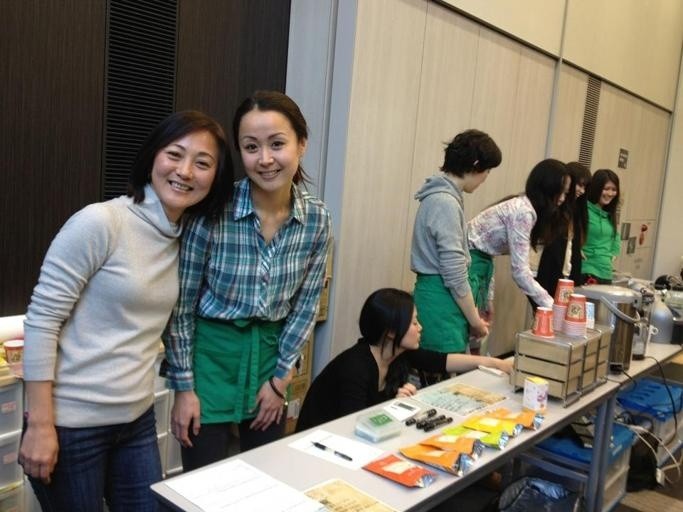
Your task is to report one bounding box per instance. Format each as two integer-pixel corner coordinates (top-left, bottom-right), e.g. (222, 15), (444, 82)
(417, 415), (445, 429)
(424, 417), (453, 432)
(406, 409), (437, 425)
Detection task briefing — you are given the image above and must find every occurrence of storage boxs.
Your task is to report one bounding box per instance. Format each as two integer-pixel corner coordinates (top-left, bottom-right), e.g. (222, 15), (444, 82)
(513, 414), (639, 511)
(284, 233), (334, 439)
(612, 376), (683, 468)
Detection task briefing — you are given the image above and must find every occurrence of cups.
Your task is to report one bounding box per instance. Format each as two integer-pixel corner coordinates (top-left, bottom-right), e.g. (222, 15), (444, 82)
(3, 339), (24, 372)
(530, 278), (596, 339)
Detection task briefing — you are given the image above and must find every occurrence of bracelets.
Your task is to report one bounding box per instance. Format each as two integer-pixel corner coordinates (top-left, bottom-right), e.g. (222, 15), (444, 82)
(267, 375), (289, 406)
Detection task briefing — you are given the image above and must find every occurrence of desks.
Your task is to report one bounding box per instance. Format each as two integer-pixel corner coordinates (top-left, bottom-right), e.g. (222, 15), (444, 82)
(148, 342), (683, 512)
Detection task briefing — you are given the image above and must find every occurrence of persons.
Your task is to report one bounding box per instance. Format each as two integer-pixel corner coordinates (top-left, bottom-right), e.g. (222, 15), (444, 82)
(528, 161), (593, 323)
(463, 158), (572, 357)
(581, 169), (621, 287)
(409, 127), (502, 384)
(294, 288), (514, 435)
(17, 108), (236, 512)
(160, 88), (332, 474)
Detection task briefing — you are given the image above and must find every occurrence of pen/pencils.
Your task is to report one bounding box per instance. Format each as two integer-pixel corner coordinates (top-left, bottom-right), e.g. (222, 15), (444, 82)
(311, 442), (352, 461)
